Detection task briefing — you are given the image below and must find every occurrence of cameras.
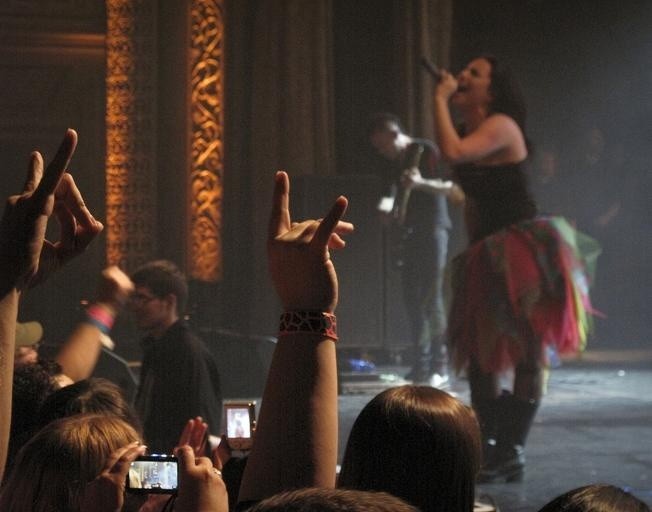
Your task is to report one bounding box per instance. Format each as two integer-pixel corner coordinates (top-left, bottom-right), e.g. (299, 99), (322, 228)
(417, 54), (441, 82)
(122, 454), (179, 495)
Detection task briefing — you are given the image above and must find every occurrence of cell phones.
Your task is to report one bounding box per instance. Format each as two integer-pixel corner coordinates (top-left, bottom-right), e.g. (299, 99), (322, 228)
(222, 401), (256, 450)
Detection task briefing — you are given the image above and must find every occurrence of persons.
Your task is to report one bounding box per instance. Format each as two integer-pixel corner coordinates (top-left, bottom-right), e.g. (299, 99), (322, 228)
(0, 123), (652, 512)
(525, 125), (651, 354)
(431, 51), (604, 486)
(360, 109), (466, 386)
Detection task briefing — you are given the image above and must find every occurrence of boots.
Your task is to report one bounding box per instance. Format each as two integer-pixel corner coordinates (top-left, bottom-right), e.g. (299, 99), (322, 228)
(470, 398), (498, 447)
(477, 396), (538, 481)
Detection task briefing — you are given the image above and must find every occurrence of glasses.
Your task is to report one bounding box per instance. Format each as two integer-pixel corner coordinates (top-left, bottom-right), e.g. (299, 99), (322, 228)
(130, 293), (159, 303)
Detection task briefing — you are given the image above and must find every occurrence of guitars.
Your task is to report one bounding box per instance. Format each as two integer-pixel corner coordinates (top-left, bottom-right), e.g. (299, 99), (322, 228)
(383, 143), (427, 242)
